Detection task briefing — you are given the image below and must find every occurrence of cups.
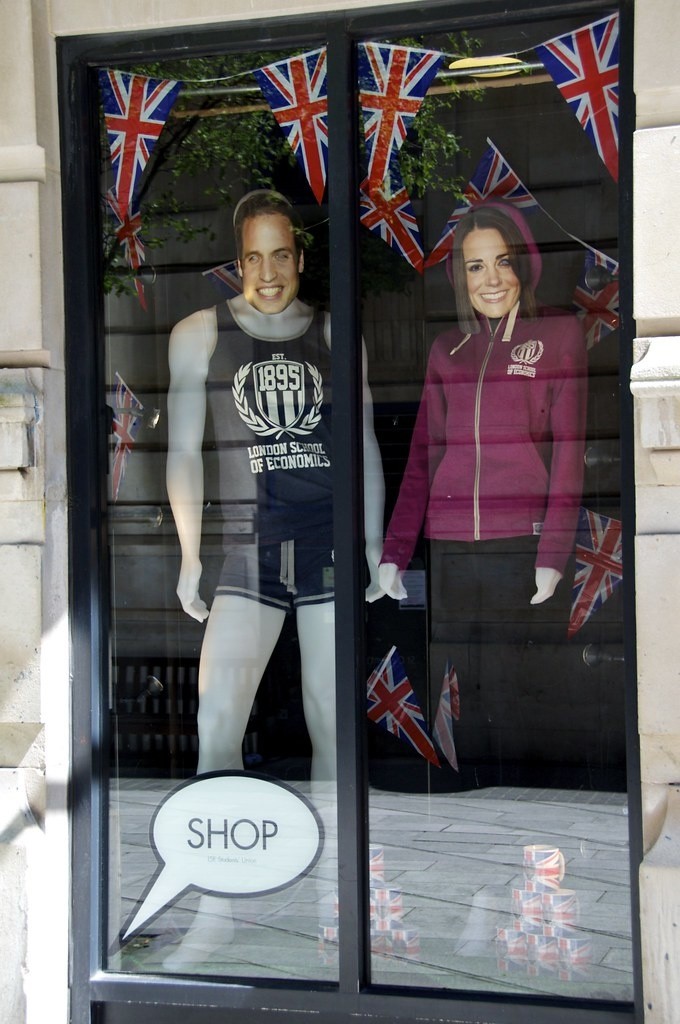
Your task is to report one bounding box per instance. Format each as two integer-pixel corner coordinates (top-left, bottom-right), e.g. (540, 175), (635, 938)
(317, 844), (422, 966)
(497, 844), (592, 981)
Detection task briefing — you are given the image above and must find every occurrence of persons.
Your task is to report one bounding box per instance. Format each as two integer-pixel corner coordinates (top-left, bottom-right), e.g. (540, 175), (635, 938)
(379, 200), (588, 957)
(162, 189), (386, 970)
